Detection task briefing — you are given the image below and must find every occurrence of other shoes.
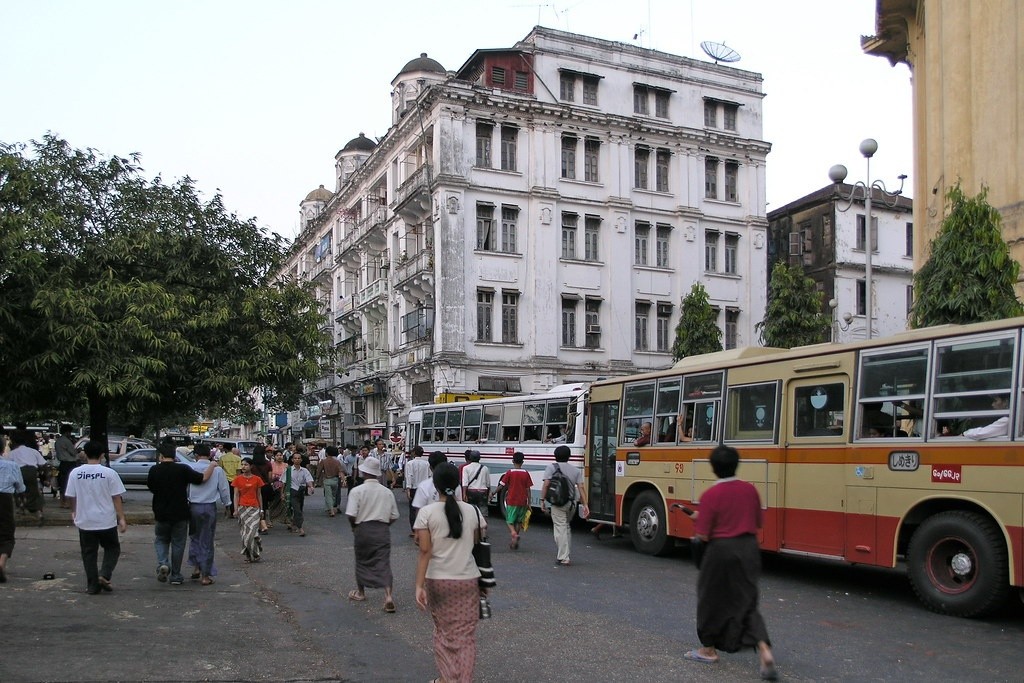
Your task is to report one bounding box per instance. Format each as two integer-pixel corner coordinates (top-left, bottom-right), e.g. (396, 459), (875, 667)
(157, 565), (169, 582)
(171, 580), (182, 585)
(0, 566), (6, 583)
(38, 515), (44, 527)
(510, 533), (520, 550)
(556, 559), (569, 565)
(254, 555), (259, 560)
(291, 527), (298, 532)
(298, 528), (304, 535)
(244, 555), (252, 563)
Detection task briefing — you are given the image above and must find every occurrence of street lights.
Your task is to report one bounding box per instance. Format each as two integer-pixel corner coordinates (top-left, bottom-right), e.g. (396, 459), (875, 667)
(825, 136), (908, 339)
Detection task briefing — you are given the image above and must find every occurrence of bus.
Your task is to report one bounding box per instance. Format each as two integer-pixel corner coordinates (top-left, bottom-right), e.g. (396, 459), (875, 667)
(401, 381), (598, 533)
(578, 309), (1024, 621)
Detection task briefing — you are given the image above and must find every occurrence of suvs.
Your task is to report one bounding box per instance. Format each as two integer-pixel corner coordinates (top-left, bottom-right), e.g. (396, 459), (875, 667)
(205, 437), (272, 465)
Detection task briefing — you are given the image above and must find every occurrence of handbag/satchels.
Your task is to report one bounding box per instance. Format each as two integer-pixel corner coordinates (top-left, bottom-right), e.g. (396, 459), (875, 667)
(480, 592), (493, 619)
(521, 510), (531, 533)
(466, 488), (489, 506)
(471, 503), (497, 587)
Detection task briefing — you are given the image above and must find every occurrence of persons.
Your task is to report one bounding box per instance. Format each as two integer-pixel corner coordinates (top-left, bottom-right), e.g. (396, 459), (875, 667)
(544, 428), (567, 443)
(345, 457), (400, 611)
(541, 445), (590, 564)
(65, 440), (126, 593)
(0, 420), (84, 586)
(179, 444), (231, 586)
(404, 446), (433, 538)
(208, 439), (406, 524)
(415, 462), (488, 683)
(492, 452), (533, 550)
(684, 445), (778, 679)
(634, 391), (1012, 447)
(412, 451), (462, 593)
(457, 450), (493, 542)
(233, 458), (263, 562)
(147, 442), (218, 584)
(250, 445), (315, 535)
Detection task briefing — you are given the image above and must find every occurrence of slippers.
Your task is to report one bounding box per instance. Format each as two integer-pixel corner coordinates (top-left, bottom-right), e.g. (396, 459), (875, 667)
(267, 522), (273, 527)
(99, 579), (113, 592)
(684, 647), (717, 664)
(191, 572), (200, 579)
(383, 602), (395, 612)
(204, 578), (216, 584)
(759, 656), (777, 678)
(260, 528), (268, 535)
(349, 589), (365, 601)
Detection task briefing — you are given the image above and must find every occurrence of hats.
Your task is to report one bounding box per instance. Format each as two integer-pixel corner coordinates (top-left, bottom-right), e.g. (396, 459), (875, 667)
(358, 456), (382, 476)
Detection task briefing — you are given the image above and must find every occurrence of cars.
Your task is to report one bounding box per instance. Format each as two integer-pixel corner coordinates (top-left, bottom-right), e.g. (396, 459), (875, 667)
(73, 438), (159, 465)
(109, 449), (197, 484)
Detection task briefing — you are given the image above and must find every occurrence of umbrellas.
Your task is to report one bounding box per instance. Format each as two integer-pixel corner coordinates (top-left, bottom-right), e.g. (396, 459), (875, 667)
(669, 502), (695, 518)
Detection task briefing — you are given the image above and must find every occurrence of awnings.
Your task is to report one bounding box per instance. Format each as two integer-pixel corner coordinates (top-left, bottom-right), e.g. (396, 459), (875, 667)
(294, 420), (320, 430)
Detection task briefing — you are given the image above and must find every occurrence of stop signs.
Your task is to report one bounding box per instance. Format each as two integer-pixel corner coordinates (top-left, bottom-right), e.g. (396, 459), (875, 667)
(389, 431), (402, 447)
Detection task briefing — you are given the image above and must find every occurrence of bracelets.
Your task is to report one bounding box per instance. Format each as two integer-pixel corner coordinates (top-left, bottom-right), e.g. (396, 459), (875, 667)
(540, 498), (544, 501)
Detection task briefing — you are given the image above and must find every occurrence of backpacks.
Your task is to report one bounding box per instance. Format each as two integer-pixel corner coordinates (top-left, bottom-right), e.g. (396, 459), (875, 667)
(544, 463), (570, 507)
